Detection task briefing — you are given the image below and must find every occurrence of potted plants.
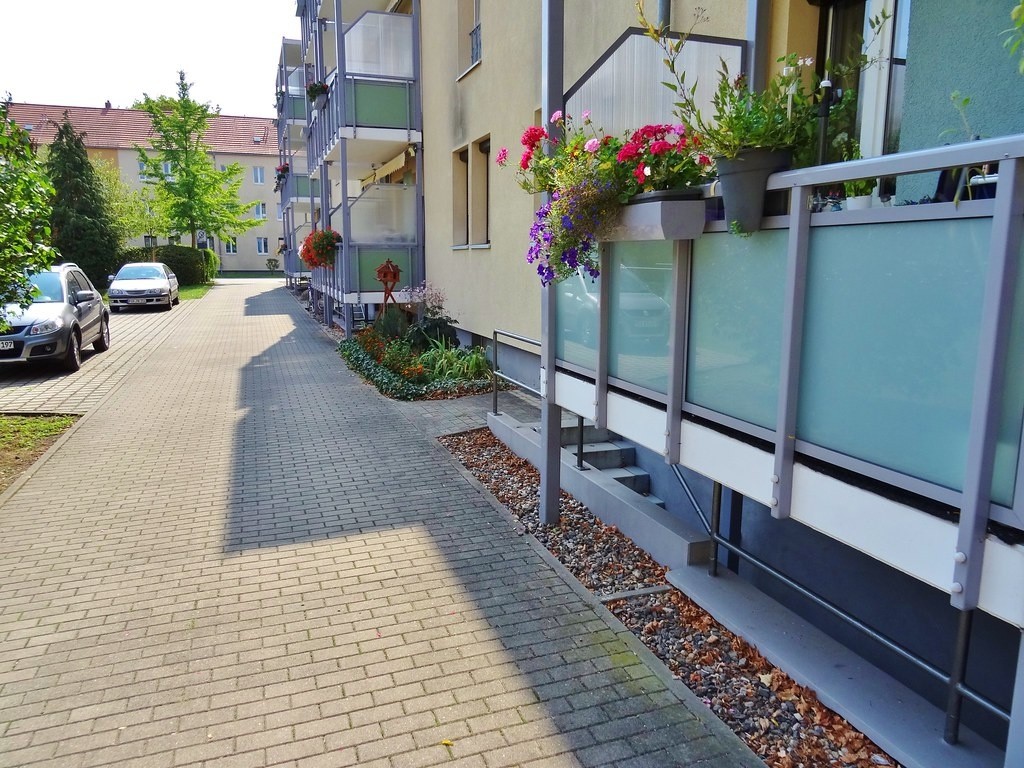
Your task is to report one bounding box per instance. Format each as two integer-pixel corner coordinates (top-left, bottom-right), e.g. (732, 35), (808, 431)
(835, 133), (877, 210)
(276, 180), (284, 185)
(274, 174), (287, 182)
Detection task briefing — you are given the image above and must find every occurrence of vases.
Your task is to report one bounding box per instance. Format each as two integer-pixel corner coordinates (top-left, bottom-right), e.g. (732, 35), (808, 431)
(284, 173), (289, 178)
(622, 188), (704, 207)
(313, 94), (327, 110)
(712, 146), (794, 233)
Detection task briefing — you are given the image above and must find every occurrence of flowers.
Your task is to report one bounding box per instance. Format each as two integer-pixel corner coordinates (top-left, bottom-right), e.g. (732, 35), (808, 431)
(306, 80), (328, 102)
(618, 123), (710, 192)
(496, 113), (616, 288)
(298, 234), (307, 260)
(275, 162), (290, 174)
(313, 226), (343, 271)
(634, 0), (901, 163)
(302, 230), (320, 270)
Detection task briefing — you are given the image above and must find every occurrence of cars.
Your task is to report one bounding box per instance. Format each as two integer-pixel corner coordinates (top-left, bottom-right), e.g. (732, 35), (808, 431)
(108, 262), (179, 312)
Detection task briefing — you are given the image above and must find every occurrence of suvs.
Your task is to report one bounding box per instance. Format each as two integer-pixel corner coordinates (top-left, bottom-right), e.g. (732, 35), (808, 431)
(0, 262), (111, 374)
